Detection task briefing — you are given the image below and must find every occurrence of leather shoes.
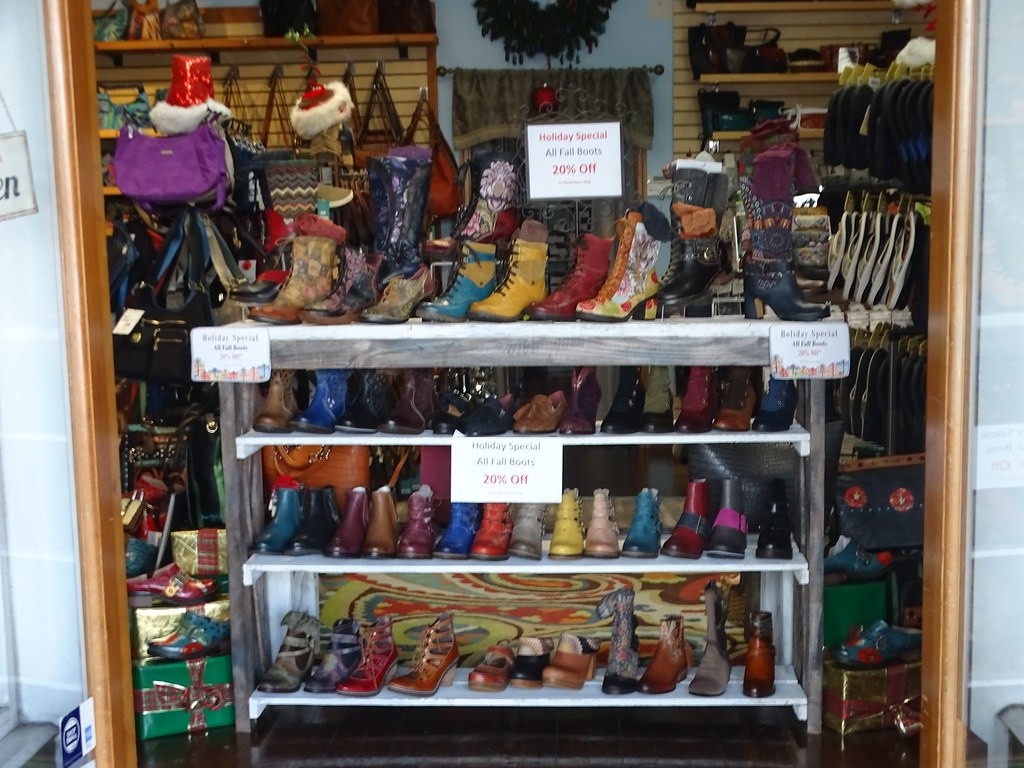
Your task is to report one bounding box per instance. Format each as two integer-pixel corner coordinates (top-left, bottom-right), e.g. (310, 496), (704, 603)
(126, 562), (222, 607)
(825, 535), (900, 580)
(147, 611), (230, 660)
(831, 619), (922, 671)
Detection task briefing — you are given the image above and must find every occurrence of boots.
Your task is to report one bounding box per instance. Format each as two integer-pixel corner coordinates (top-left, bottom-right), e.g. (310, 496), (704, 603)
(229, 119), (832, 325)
(255, 368), (798, 436)
(252, 472), (791, 560)
(258, 580), (777, 698)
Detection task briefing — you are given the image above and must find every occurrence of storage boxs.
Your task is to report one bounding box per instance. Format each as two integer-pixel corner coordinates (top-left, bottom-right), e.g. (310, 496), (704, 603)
(129, 592), (231, 659)
(823, 658), (923, 731)
(171, 527), (230, 575)
(130, 653), (237, 737)
(823, 579), (891, 649)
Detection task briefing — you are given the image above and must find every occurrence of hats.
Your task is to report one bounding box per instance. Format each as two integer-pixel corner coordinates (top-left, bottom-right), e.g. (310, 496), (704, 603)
(788, 49), (823, 73)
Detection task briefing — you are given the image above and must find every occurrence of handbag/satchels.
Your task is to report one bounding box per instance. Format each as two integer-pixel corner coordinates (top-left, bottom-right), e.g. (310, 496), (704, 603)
(778, 103), (829, 130)
(222, 64), (466, 258)
(315, 1), (379, 36)
(104, 202), (293, 575)
(113, 109), (235, 214)
(92, 1), (128, 41)
(160, 0), (205, 40)
(719, 28), (787, 73)
(96, 81), (151, 129)
(375, 1), (436, 35)
(822, 42), (869, 71)
(123, 0), (162, 41)
(688, 19), (747, 80)
(259, 0), (318, 38)
(696, 88), (740, 136)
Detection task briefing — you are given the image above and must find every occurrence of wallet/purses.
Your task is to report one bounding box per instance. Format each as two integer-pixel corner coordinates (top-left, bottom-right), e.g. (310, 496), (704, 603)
(750, 99), (785, 123)
(716, 109), (757, 131)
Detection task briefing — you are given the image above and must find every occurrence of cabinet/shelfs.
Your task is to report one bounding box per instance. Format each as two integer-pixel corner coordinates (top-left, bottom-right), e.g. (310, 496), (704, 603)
(695, 3), (897, 140)
(94, 34), (440, 192)
(208, 324), (827, 738)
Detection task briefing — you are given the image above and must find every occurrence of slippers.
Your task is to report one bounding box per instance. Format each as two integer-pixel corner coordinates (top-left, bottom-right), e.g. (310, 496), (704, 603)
(827, 74), (937, 463)
